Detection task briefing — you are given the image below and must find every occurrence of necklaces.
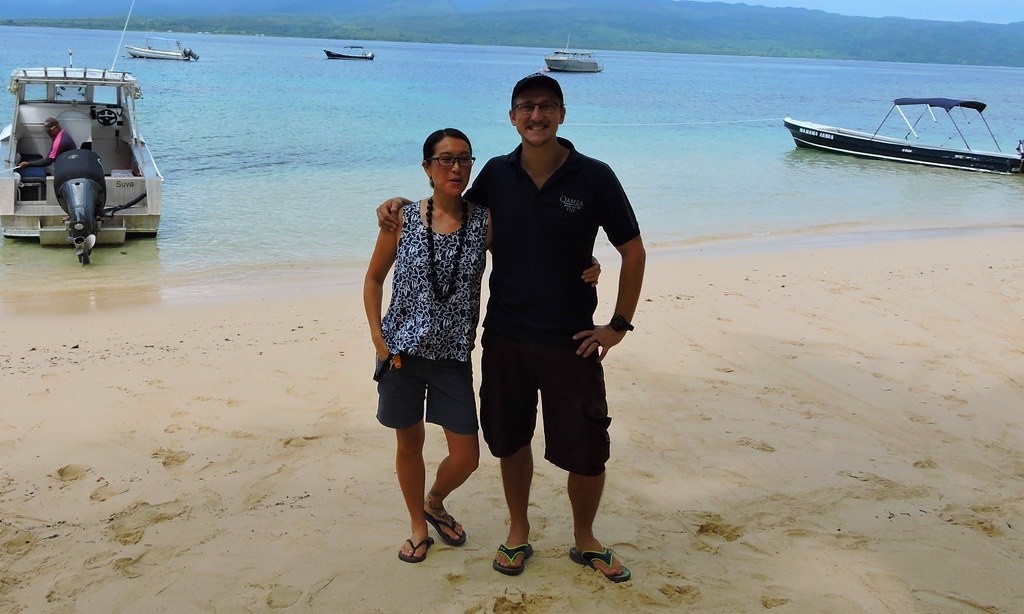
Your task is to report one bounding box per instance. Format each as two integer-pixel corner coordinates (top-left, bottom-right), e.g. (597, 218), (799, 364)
(427, 199), (468, 303)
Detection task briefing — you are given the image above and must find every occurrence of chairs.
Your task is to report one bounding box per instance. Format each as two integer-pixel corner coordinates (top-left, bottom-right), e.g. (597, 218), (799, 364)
(17, 152), (46, 201)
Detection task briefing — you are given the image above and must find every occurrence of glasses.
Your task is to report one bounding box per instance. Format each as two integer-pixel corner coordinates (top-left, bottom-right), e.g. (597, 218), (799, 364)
(512, 100), (564, 113)
(428, 155), (476, 167)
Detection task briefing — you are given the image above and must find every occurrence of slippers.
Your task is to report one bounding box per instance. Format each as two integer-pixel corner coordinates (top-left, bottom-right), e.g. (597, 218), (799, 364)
(570, 546), (631, 583)
(398, 536), (435, 563)
(423, 507), (467, 548)
(493, 543), (534, 576)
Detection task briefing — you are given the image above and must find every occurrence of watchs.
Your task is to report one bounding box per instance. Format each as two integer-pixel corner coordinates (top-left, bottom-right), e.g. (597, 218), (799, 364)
(610, 315), (634, 332)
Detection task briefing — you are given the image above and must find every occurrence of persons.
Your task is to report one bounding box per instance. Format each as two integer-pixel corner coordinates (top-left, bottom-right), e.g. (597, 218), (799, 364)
(363, 127), (601, 564)
(19, 117), (77, 177)
(376, 71), (646, 583)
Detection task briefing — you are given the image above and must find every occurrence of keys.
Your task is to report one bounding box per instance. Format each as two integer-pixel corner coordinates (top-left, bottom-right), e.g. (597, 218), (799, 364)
(389, 359), (395, 371)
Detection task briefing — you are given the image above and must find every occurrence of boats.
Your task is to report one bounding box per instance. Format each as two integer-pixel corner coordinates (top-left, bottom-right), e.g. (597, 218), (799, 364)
(125, 39), (191, 61)
(0, 65), (165, 248)
(544, 34), (605, 72)
(783, 96), (1024, 175)
(323, 45), (375, 60)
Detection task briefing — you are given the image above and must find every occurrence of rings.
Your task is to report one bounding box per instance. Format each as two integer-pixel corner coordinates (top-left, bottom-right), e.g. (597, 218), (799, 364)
(595, 341), (600, 346)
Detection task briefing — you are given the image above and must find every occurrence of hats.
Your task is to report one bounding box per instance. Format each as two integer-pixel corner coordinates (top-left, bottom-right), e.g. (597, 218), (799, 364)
(511, 71), (564, 100)
